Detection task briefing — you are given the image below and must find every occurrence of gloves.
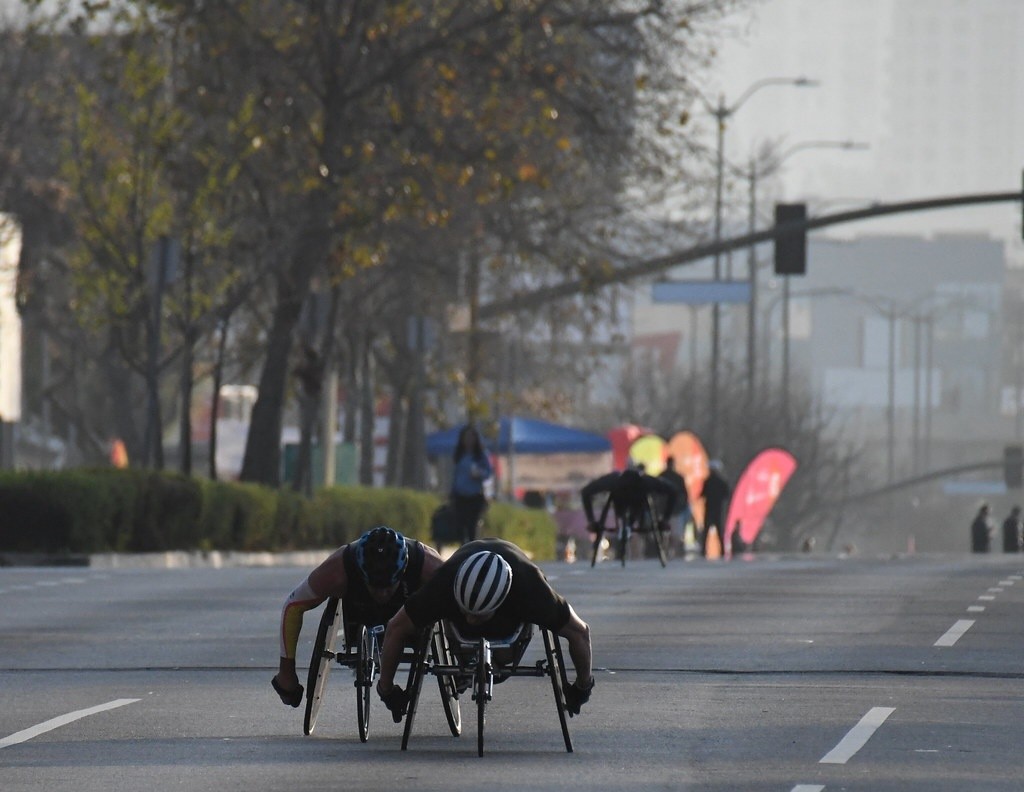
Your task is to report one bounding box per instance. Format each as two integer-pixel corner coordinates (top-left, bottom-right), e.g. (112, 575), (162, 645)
(376, 681), (406, 723)
(564, 677), (594, 714)
(269, 675), (304, 708)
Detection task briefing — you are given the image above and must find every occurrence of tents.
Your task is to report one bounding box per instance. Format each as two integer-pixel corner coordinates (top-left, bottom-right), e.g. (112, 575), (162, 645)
(425, 416), (611, 454)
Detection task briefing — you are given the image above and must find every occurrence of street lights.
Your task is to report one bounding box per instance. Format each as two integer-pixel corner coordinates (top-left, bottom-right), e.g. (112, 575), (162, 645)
(693, 137), (958, 554)
(668, 71), (820, 463)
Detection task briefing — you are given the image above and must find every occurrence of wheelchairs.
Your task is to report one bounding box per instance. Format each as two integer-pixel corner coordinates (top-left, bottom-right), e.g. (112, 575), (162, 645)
(303, 580), (573, 757)
(588, 489), (670, 569)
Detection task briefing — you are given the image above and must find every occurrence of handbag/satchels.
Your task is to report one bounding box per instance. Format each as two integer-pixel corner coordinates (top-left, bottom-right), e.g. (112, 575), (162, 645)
(431, 506), (464, 544)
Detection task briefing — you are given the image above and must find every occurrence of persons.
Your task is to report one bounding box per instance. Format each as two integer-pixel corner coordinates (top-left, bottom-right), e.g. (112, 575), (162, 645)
(269, 526), (445, 708)
(699, 459), (730, 559)
(377, 537), (594, 714)
(450, 426), (489, 545)
(657, 456), (687, 561)
(971, 504), (994, 553)
(1003, 506), (1020, 552)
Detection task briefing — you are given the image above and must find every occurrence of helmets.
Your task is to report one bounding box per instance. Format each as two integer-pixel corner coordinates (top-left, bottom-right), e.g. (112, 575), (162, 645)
(453, 550), (512, 615)
(357, 526), (408, 588)
